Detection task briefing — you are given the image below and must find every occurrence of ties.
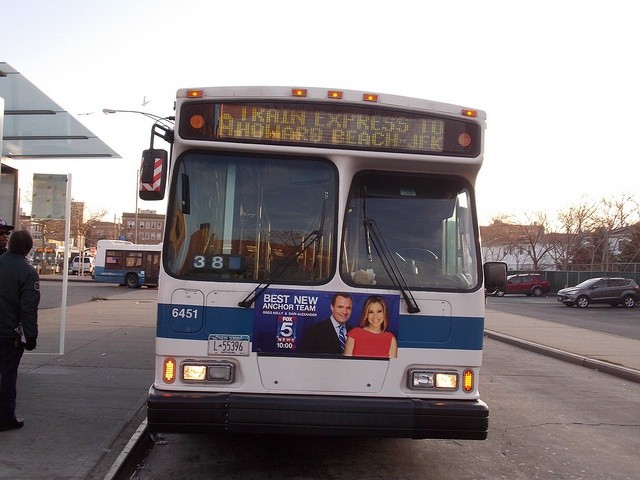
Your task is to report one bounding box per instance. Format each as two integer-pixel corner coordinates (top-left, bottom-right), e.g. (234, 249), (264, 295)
(339, 325), (345, 352)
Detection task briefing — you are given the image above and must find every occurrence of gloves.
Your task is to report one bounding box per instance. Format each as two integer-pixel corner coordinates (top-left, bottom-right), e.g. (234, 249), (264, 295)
(22, 339), (37, 350)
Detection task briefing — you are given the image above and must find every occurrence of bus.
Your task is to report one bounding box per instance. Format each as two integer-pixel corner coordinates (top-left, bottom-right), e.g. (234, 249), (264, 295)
(93, 237), (163, 288)
(138, 83), (490, 442)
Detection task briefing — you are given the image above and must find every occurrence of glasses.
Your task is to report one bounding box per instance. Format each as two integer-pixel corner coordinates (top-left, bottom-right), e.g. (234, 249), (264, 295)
(0, 230), (11, 235)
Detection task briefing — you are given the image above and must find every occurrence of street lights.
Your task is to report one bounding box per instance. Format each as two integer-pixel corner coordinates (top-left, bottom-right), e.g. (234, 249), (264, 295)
(101, 106), (178, 139)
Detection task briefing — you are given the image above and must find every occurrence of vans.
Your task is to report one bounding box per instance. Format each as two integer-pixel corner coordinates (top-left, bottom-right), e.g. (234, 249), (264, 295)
(69, 255), (94, 277)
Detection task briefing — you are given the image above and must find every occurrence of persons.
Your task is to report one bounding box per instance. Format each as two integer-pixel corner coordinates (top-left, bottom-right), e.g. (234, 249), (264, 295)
(0, 219), (15, 255)
(343, 295), (397, 358)
(0, 230), (41, 431)
(301, 293), (354, 354)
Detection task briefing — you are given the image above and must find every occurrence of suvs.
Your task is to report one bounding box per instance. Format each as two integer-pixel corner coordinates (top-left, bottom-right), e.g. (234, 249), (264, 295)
(486, 273), (552, 298)
(555, 277), (639, 317)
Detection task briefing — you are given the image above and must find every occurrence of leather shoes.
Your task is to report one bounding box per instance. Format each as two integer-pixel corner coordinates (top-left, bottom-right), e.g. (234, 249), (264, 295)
(1, 417), (25, 432)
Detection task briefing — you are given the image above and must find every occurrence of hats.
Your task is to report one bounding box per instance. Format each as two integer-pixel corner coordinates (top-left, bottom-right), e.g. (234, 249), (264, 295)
(0, 220), (15, 231)
